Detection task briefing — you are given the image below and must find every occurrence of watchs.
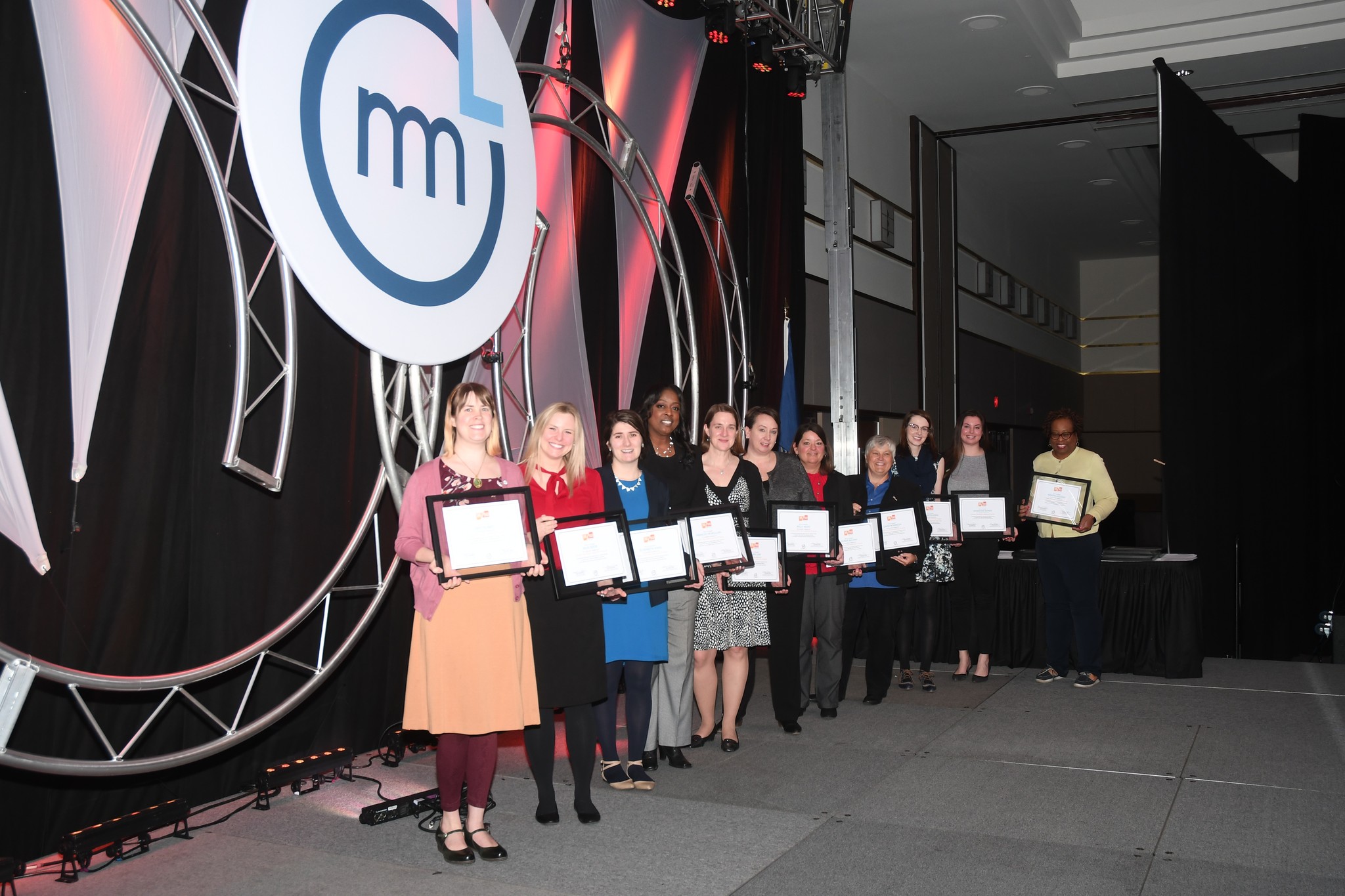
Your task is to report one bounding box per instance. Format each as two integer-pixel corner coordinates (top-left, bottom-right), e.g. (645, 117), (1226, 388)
(1090, 515), (1096, 524)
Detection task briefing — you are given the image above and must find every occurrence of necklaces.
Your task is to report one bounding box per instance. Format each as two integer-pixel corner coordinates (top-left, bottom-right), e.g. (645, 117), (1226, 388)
(652, 437), (673, 457)
(709, 454), (729, 474)
(455, 452), (485, 488)
(614, 467), (642, 492)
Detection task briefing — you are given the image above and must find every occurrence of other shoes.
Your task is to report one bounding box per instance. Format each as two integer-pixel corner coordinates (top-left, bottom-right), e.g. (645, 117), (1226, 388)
(535, 802), (560, 825)
(820, 708), (837, 719)
(799, 708), (807, 716)
(574, 800), (600, 825)
(862, 696), (882, 705)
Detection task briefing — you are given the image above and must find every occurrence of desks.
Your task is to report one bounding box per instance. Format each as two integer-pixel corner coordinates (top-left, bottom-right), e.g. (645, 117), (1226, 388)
(852, 548), (1205, 679)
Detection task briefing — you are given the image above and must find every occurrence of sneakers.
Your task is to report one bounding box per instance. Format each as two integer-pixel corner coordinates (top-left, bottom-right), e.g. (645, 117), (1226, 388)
(1074, 672), (1100, 688)
(1036, 666), (1064, 683)
(898, 669), (916, 690)
(918, 669), (937, 692)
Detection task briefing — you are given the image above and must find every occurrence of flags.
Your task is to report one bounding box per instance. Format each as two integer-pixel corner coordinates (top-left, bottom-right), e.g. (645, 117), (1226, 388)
(779, 317), (800, 454)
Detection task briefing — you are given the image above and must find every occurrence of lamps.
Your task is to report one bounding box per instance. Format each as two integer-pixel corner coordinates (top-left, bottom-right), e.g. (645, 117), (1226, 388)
(254, 745), (352, 809)
(745, 26), (774, 72)
(785, 56), (810, 98)
(57, 798), (193, 883)
(703, 0), (735, 45)
(383, 728), (437, 767)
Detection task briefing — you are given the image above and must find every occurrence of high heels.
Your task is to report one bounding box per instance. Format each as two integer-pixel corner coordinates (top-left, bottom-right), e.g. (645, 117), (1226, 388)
(658, 745), (692, 768)
(642, 748), (659, 771)
(688, 722), (717, 748)
(972, 662), (991, 683)
(952, 656), (973, 681)
(721, 729), (740, 752)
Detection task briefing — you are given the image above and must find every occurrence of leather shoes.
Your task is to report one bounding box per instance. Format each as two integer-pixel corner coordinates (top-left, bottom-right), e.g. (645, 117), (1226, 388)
(626, 760), (655, 791)
(463, 818), (508, 862)
(600, 759), (634, 790)
(716, 719), (743, 733)
(777, 720), (802, 735)
(809, 694), (843, 704)
(435, 818), (475, 864)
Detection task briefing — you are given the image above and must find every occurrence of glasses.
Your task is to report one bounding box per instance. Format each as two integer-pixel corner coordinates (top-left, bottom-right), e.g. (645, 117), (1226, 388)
(907, 424), (930, 434)
(1048, 430), (1076, 440)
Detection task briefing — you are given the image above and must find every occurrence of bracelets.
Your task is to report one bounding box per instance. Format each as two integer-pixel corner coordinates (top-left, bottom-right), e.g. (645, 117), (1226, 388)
(952, 523), (956, 525)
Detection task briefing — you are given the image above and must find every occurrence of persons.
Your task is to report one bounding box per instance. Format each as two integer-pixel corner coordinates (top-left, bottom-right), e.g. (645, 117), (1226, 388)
(638, 381), (748, 771)
(394, 381), (549, 864)
(1019, 407), (1118, 688)
(518, 402), (627, 825)
(888, 410), (964, 692)
(809, 435), (932, 705)
(688, 403), (791, 752)
(939, 409), (1018, 684)
(591, 409), (705, 790)
(779, 422), (853, 723)
(722, 406), (844, 735)
